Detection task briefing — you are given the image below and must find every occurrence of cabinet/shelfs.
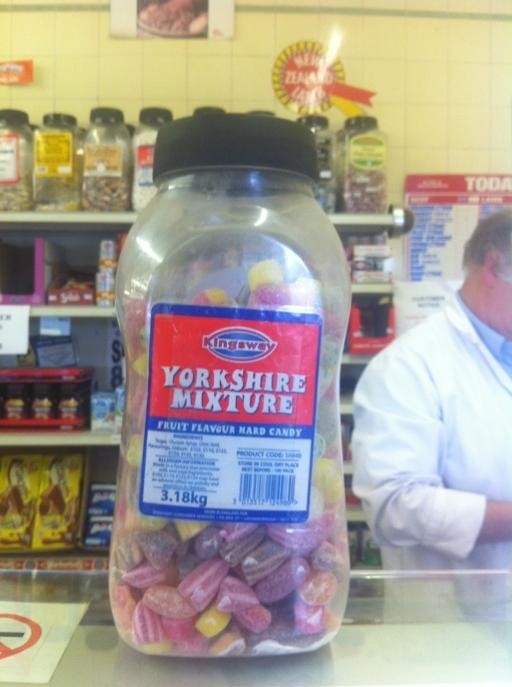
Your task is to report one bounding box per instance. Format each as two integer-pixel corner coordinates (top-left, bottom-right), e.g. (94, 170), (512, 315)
(0, 212), (396, 557)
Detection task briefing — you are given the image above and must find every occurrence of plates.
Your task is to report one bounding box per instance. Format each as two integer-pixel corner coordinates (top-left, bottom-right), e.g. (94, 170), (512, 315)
(136, 10), (208, 37)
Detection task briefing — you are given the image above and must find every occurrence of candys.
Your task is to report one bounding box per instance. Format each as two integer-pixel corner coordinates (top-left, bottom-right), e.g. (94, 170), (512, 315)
(108, 257), (349, 659)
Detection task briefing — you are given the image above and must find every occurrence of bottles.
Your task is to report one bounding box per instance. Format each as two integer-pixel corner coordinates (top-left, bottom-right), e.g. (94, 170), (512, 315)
(0, 105), (390, 214)
(107, 111), (354, 660)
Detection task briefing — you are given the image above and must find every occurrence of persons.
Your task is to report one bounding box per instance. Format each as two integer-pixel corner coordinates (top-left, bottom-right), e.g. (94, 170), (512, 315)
(345, 204), (512, 570)
